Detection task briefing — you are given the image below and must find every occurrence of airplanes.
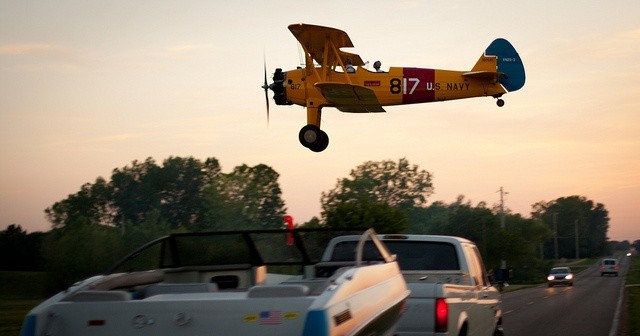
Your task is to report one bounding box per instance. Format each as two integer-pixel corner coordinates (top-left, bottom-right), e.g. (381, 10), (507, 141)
(260, 21), (527, 152)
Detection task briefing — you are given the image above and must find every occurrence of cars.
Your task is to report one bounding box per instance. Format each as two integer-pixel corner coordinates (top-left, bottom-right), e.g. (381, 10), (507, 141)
(547, 265), (576, 286)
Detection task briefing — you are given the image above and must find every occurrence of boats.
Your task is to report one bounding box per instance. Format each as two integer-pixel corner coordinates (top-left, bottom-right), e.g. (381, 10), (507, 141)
(20, 211), (413, 335)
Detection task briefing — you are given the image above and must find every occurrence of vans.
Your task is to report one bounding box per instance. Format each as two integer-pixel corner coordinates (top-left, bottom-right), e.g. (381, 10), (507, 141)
(600, 257), (620, 276)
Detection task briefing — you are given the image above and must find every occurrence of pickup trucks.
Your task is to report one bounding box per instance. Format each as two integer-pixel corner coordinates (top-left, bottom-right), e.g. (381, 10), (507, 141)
(318, 232), (506, 335)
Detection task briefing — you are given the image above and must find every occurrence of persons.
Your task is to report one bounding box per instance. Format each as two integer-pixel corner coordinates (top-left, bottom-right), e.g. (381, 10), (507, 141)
(343, 57), (355, 72)
(373, 61), (385, 72)
